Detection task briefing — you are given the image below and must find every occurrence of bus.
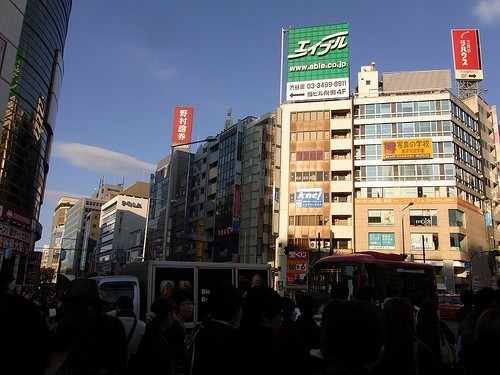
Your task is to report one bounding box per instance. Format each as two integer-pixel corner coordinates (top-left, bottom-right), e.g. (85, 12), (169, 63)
(301, 251), (439, 330)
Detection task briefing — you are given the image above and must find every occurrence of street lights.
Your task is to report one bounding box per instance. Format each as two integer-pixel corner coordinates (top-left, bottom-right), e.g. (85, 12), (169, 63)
(401, 202), (415, 258)
(160, 134), (219, 261)
(141, 197), (178, 263)
(58, 237), (79, 276)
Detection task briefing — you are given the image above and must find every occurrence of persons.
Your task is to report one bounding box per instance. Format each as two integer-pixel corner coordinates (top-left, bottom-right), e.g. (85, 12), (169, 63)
(0, 270), (196, 375)
(323, 281), (500, 375)
(187, 284), (321, 375)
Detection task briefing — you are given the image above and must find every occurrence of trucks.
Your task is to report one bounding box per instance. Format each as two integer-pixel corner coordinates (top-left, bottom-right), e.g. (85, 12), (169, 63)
(79, 258), (276, 346)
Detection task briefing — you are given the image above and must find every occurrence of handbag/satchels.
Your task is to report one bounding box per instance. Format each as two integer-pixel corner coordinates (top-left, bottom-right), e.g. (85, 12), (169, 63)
(437, 319), (456, 364)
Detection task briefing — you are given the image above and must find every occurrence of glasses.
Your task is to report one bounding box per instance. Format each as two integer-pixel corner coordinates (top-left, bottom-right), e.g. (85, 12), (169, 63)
(278, 312), (283, 318)
(179, 301), (196, 306)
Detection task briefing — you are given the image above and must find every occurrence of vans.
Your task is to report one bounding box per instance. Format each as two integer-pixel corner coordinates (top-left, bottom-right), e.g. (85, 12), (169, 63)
(437, 293), (461, 316)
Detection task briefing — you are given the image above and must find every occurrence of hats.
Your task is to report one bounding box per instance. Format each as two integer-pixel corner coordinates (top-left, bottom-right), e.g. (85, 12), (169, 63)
(112, 296), (134, 310)
(64, 278), (109, 304)
